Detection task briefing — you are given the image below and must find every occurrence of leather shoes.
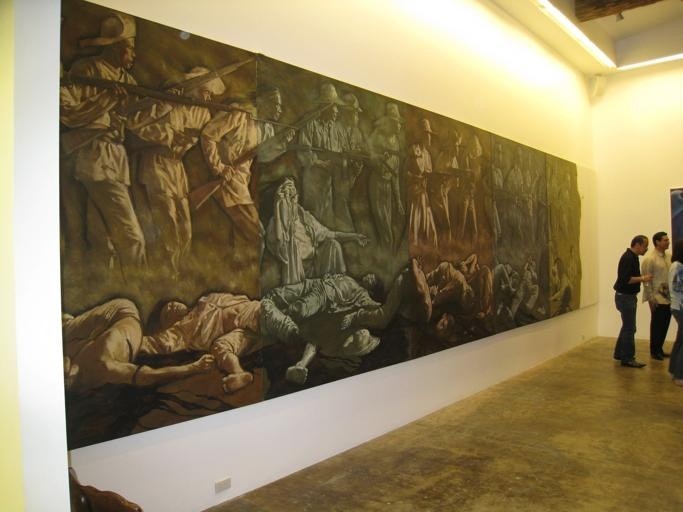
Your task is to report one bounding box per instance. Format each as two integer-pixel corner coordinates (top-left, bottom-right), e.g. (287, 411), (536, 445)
(612, 354), (647, 370)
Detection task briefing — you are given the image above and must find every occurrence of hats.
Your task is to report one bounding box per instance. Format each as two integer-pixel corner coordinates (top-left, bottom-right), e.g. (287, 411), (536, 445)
(413, 118), (439, 137)
(181, 64), (228, 97)
(311, 82), (347, 108)
(338, 92), (363, 115)
(382, 100), (406, 124)
(441, 126), (463, 148)
(76, 13), (137, 46)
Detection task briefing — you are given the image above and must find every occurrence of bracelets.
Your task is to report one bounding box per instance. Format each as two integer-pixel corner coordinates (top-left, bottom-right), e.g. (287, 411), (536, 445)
(640, 274), (645, 282)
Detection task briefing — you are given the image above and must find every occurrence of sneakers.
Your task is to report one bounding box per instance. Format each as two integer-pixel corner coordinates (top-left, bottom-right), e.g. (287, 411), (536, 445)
(649, 351), (672, 362)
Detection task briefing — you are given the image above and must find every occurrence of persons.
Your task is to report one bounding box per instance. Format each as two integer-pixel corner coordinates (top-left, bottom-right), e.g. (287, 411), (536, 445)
(610, 235), (653, 368)
(667, 237), (683, 388)
(642, 231), (673, 361)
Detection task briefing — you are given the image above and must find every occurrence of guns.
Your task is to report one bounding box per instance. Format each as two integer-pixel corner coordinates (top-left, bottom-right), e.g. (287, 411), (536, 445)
(312, 144), (424, 175)
(60, 56), (257, 155)
(60, 74), (300, 131)
(188, 103), (325, 210)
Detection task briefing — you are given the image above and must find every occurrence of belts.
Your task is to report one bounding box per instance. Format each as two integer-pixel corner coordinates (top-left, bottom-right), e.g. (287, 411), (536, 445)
(140, 140), (184, 162)
